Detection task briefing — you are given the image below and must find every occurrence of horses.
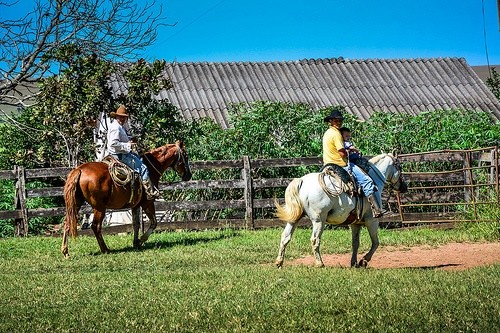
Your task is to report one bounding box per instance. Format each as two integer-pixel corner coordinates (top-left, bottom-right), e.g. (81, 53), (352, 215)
(271, 148), (408, 268)
(61, 140), (192, 259)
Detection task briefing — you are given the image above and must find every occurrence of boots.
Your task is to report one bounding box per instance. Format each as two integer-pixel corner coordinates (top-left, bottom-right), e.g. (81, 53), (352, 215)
(368, 194), (387, 218)
(142, 179), (159, 200)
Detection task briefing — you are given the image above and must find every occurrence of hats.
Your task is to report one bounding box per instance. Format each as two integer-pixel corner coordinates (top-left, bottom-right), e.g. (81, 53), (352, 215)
(324, 110), (345, 122)
(110, 106), (129, 116)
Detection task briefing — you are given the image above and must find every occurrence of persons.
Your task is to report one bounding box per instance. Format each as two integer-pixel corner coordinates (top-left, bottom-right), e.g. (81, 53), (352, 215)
(106, 106), (162, 199)
(322, 109), (387, 218)
